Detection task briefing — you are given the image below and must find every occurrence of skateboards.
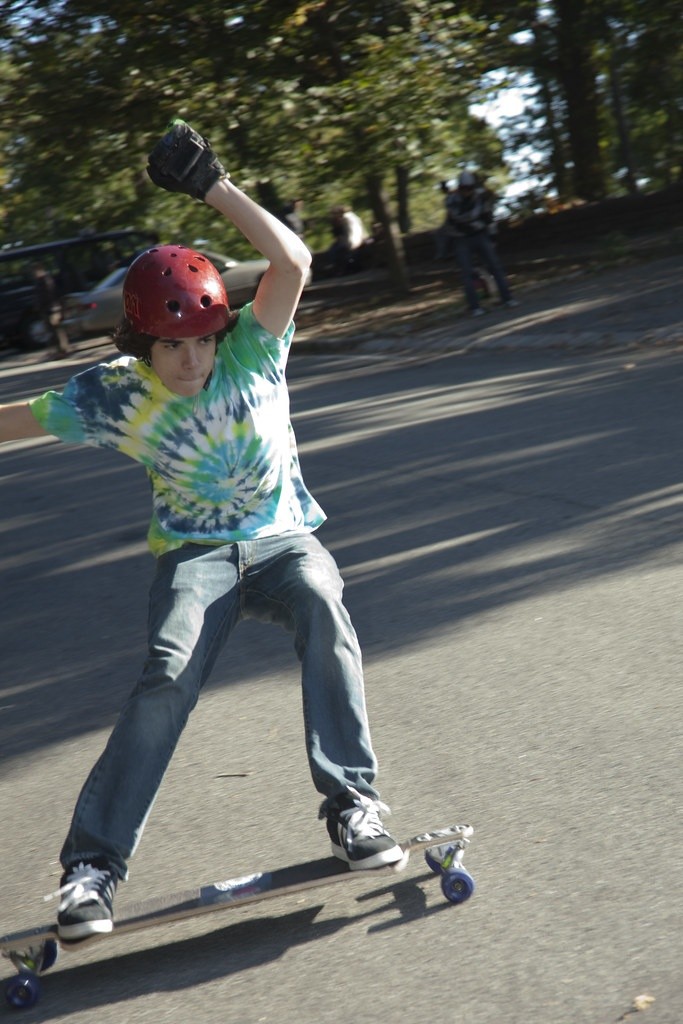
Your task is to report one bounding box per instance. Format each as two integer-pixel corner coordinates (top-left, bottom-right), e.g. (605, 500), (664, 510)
(0, 823), (478, 1007)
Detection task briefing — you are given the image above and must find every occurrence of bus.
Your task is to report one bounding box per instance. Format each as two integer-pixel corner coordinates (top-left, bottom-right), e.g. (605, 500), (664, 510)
(0, 230), (164, 350)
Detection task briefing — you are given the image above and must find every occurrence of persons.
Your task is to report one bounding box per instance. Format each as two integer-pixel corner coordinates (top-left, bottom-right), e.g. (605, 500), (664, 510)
(27, 262), (71, 361)
(0, 121), (402, 939)
(286, 160), (523, 316)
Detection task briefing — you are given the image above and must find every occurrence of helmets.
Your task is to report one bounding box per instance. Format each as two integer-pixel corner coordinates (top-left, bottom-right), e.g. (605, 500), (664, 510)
(484, 176), (506, 197)
(122, 245), (230, 338)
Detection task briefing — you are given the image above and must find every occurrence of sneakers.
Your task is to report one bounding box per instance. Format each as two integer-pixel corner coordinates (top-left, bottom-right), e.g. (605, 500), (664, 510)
(319, 785), (403, 870)
(43, 862), (118, 940)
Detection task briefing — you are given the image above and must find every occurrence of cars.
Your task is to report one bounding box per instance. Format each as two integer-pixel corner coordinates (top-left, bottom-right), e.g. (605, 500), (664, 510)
(81, 249), (312, 337)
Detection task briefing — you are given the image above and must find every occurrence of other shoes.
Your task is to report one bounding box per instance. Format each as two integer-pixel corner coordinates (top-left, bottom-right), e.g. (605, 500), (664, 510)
(505, 300), (521, 307)
(473, 308), (485, 317)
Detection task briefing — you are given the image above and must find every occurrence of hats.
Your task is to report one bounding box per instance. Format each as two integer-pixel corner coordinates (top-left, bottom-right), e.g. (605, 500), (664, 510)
(459, 172), (475, 188)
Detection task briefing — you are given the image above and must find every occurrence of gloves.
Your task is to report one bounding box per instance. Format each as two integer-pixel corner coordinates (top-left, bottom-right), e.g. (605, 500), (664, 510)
(146, 119), (225, 199)
